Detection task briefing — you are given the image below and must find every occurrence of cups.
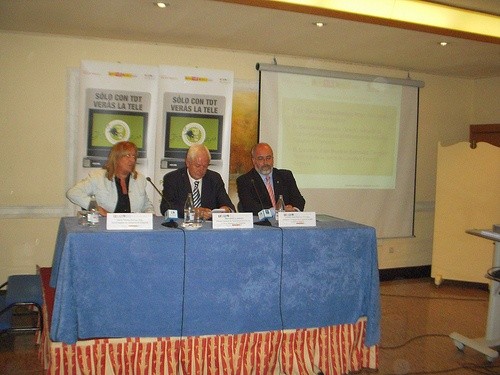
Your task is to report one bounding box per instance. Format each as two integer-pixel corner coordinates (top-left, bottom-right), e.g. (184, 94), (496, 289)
(78, 210), (91, 226)
(195, 208), (205, 223)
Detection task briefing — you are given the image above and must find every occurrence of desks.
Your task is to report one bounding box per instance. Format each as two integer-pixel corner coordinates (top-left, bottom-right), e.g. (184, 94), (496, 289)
(446, 229), (500, 364)
(50, 214), (380, 346)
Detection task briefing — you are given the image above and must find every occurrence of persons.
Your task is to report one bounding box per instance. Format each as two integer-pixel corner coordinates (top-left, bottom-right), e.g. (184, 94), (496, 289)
(67, 141), (159, 215)
(160, 145), (238, 221)
(238, 143), (306, 217)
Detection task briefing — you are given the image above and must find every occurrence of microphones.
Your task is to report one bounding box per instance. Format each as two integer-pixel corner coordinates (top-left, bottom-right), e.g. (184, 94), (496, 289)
(146, 176), (179, 228)
(251, 178), (272, 225)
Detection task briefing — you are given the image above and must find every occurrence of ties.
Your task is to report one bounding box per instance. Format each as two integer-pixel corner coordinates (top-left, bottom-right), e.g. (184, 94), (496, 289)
(265, 175), (276, 207)
(192, 181), (202, 209)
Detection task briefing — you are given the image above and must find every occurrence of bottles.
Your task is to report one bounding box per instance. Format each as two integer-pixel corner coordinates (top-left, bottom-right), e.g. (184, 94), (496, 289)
(285, 205), (295, 212)
(183, 193), (195, 227)
(275, 194), (285, 227)
(88, 194), (99, 227)
